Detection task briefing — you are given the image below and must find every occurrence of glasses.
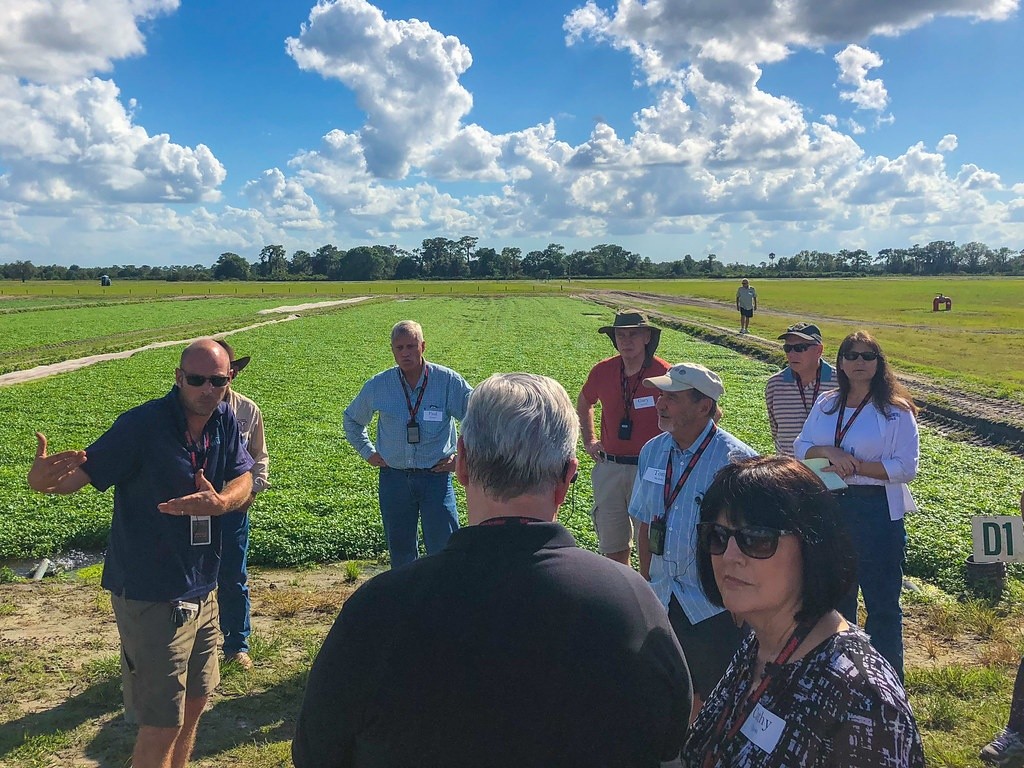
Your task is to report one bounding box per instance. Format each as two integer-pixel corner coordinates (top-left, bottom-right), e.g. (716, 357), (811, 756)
(180, 367), (231, 387)
(782, 341), (818, 353)
(695, 521), (799, 559)
(843, 351), (878, 361)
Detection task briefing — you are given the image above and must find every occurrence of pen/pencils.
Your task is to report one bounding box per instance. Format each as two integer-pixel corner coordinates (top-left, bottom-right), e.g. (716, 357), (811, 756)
(850, 447), (857, 479)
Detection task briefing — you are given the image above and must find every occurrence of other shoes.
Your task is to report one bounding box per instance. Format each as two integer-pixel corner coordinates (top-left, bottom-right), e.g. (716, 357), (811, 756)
(739, 328), (750, 334)
(227, 648), (251, 671)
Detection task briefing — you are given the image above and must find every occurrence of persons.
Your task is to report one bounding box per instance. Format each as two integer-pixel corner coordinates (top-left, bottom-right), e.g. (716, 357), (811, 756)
(793, 331), (919, 685)
(628, 363), (760, 729)
(735, 279), (757, 333)
(980, 490), (1024, 765)
(344, 320), (473, 568)
(25, 339), (256, 768)
(766, 321), (839, 460)
(578, 308), (722, 579)
(680, 455), (927, 768)
(291, 372), (693, 768)
(215, 340), (270, 668)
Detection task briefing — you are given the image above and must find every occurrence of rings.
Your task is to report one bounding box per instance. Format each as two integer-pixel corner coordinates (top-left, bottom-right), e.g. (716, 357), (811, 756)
(181, 510), (183, 515)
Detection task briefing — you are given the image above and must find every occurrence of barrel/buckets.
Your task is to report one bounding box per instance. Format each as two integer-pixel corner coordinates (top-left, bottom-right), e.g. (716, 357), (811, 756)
(965, 555), (1007, 592)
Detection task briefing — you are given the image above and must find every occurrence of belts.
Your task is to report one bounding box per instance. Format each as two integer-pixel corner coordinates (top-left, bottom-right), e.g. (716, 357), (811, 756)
(599, 451), (639, 465)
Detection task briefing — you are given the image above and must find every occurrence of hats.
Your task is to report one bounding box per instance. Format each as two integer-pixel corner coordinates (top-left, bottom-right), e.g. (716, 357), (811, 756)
(598, 311), (661, 356)
(777, 322), (822, 344)
(641, 362), (724, 402)
(213, 341), (250, 371)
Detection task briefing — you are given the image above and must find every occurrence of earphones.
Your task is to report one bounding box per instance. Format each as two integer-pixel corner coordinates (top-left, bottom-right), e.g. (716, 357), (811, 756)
(571, 472), (579, 483)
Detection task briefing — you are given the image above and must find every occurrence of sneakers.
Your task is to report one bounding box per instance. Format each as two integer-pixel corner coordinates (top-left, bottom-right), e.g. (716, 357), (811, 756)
(978, 729), (1024, 765)
